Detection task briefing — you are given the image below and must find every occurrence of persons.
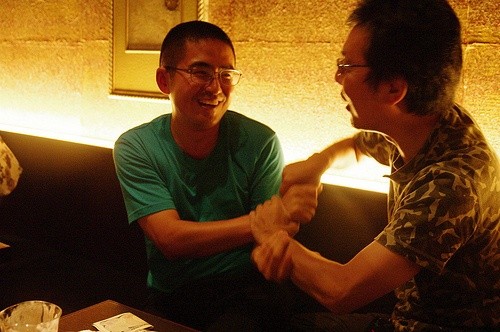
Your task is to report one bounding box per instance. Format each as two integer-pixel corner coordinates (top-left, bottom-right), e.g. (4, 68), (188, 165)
(112, 20), (323, 332)
(248, 0), (500, 332)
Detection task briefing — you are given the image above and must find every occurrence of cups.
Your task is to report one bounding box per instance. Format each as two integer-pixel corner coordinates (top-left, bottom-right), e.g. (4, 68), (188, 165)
(0, 300), (62, 332)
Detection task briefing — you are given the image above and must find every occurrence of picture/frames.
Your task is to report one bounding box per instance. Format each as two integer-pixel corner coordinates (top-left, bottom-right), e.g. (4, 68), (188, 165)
(107, 0), (211, 101)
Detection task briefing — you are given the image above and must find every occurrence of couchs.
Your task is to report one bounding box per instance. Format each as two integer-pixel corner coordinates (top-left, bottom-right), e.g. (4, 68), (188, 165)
(0, 131), (389, 332)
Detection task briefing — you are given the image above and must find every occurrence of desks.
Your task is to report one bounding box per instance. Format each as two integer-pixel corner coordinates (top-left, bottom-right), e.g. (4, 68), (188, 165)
(58, 299), (199, 332)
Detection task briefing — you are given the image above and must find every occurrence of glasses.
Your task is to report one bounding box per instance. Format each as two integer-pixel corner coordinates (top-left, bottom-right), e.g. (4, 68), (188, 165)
(161, 63), (244, 87)
(335, 57), (370, 75)
